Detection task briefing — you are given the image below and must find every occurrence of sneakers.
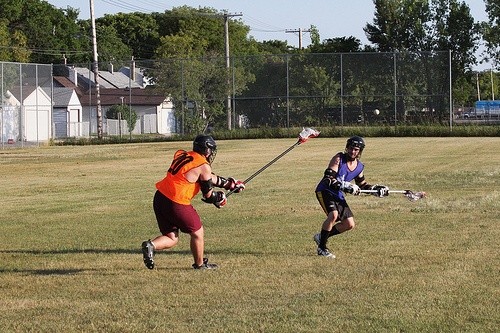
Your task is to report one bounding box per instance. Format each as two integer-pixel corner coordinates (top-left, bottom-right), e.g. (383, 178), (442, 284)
(314, 234), (321, 246)
(141, 239), (154, 270)
(317, 248), (336, 258)
(192, 258), (219, 269)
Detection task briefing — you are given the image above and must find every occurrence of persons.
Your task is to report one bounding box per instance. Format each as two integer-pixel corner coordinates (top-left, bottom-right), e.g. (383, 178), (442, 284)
(313, 136), (390, 258)
(141, 133), (246, 271)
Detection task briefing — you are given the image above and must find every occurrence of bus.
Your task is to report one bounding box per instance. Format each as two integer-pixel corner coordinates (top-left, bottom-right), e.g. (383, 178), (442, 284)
(475, 100), (500, 119)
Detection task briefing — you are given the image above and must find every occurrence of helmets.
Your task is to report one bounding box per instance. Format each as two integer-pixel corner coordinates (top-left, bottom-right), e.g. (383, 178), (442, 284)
(346, 136), (365, 153)
(193, 136), (218, 164)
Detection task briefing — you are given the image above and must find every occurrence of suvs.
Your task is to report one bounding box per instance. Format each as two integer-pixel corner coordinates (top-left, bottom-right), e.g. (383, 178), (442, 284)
(461, 109), (484, 119)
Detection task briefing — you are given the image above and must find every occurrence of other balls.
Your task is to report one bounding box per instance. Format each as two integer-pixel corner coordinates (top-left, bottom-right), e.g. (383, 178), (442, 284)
(374, 109), (380, 115)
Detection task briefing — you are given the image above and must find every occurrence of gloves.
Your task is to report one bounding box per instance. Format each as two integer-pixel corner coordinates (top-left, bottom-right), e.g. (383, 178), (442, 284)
(372, 184), (389, 198)
(225, 178), (246, 193)
(202, 190), (227, 209)
(345, 183), (362, 196)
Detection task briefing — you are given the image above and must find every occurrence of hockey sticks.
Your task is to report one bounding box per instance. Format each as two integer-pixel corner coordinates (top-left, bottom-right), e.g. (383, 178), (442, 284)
(344, 187), (427, 201)
(224, 126), (321, 198)
